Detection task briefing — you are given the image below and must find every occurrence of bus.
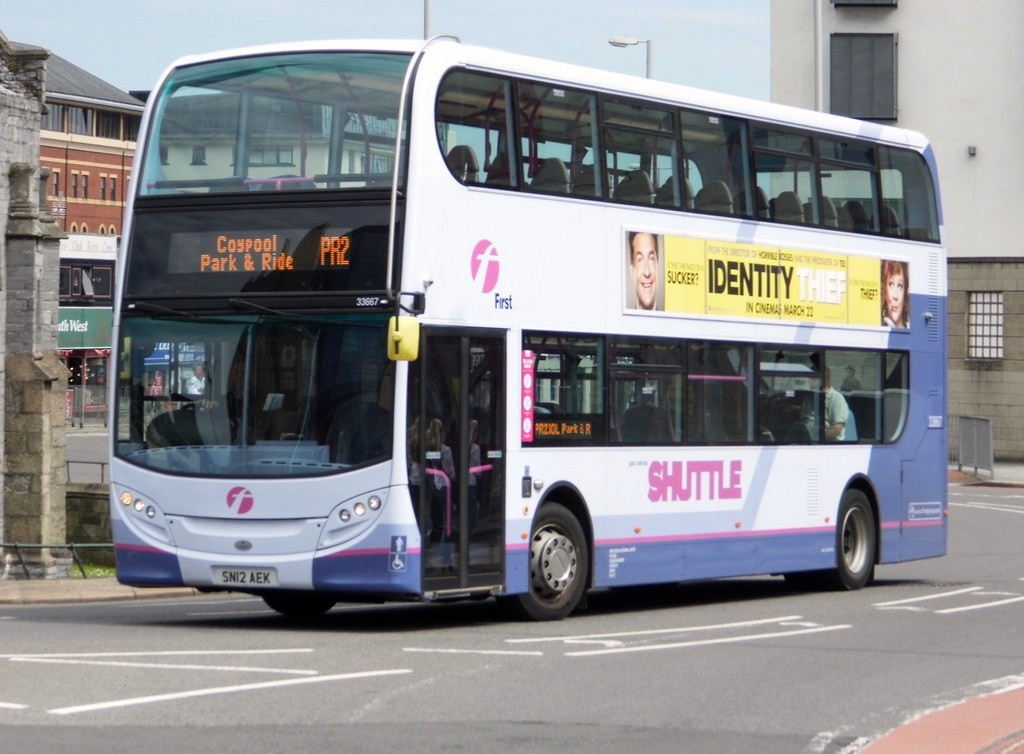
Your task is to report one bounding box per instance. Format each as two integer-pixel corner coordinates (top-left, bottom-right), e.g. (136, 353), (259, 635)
(106, 34), (951, 621)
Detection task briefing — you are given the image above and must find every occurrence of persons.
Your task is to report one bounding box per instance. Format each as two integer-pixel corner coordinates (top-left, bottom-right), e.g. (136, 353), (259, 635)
(150, 376), (163, 415)
(880, 260), (910, 330)
(187, 365), (206, 395)
(840, 364), (861, 392)
(199, 344), (265, 437)
(628, 231), (662, 310)
(407, 414), (481, 571)
(799, 365), (859, 442)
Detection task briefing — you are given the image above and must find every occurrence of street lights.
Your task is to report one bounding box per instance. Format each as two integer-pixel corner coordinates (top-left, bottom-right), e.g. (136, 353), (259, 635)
(609, 37), (653, 78)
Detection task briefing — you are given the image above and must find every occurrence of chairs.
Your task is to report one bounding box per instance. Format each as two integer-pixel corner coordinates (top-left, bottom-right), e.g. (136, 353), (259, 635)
(845, 201), (870, 232)
(837, 208), (853, 230)
(485, 152), (507, 184)
(735, 185), (770, 219)
(774, 191), (805, 224)
(783, 422), (812, 441)
(698, 181), (734, 214)
(870, 206), (901, 236)
(655, 176), (694, 209)
(693, 426), (726, 442)
(446, 145), (480, 182)
(823, 196), (838, 228)
(739, 424), (776, 441)
(803, 202), (813, 223)
(531, 157), (571, 193)
(613, 169), (655, 203)
(573, 165), (612, 198)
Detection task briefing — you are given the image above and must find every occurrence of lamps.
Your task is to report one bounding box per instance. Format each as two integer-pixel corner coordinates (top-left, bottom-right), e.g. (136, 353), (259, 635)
(968, 146), (977, 157)
